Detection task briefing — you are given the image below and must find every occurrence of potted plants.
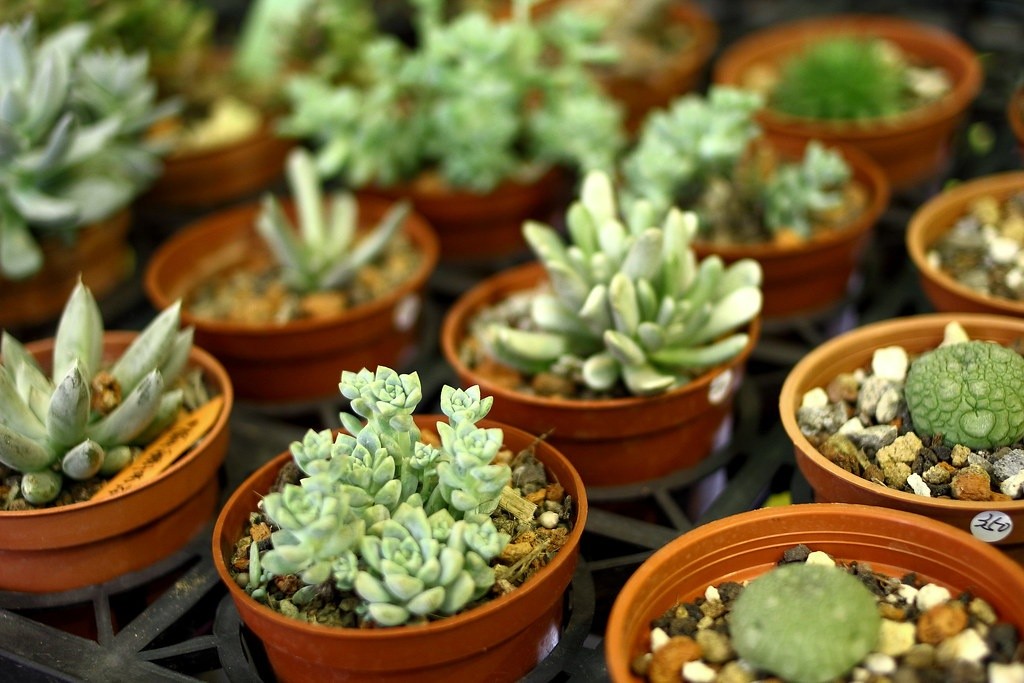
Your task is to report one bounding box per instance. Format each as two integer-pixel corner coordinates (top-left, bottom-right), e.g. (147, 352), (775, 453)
(0, 0), (1024, 683)
